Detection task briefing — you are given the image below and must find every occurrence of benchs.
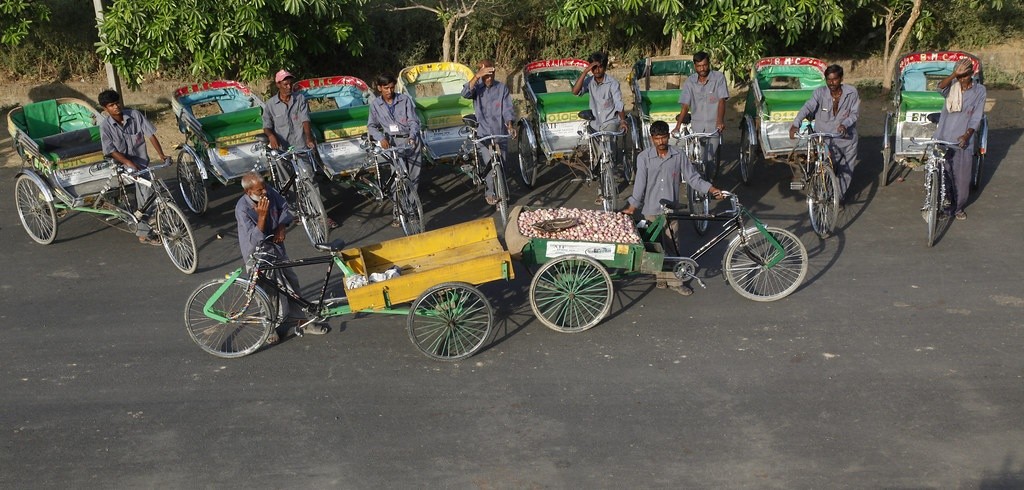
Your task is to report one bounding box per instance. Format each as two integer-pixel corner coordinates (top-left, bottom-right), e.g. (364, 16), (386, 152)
(897, 91), (944, 141)
(413, 93), (475, 130)
(198, 106), (264, 152)
(308, 104), (370, 145)
(536, 91), (591, 124)
(761, 89), (813, 134)
(639, 90), (689, 125)
(32, 125), (105, 171)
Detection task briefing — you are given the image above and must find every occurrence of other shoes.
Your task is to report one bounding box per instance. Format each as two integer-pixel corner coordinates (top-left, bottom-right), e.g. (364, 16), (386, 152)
(954, 211), (967, 220)
(392, 216), (402, 228)
(320, 215), (338, 228)
(495, 202), (509, 211)
(938, 214), (950, 221)
(670, 285), (693, 296)
(293, 214), (303, 226)
(484, 190), (498, 205)
(408, 214), (418, 224)
(655, 281), (667, 289)
(595, 196), (603, 206)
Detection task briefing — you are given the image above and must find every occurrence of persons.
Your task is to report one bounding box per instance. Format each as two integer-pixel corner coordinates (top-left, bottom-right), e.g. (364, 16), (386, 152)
(790, 63), (860, 212)
(262, 69), (339, 228)
(621, 120), (723, 296)
(98, 86), (171, 245)
(235, 170), (327, 343)
(368, 71), (422, 227)
(462, 59), (516, 211)
(572, 51), (628, 205)
(931, 59), (986, 220)
(671, 50), (729, 187)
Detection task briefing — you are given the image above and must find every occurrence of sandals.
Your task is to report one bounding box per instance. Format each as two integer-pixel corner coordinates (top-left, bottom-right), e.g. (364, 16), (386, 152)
(140, 234), (163, 246)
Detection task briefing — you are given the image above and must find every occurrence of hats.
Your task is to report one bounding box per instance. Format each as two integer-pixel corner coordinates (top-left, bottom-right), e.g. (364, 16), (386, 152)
(275, 69), (294, 83)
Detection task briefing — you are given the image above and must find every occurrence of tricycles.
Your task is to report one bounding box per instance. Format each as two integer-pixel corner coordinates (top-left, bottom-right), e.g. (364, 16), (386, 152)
(503, 190), (810, 335)
(7, 97), (198, 274)
(171, 55), (732, 251)
(738, 57), (846, 238)
(879, 52), (989, 247)
(184, 216), (516, 362)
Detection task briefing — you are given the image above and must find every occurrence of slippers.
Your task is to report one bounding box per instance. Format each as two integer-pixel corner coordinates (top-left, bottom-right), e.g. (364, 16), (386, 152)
(264, 327), (280, 345)
(298, 322), (328, 335)
